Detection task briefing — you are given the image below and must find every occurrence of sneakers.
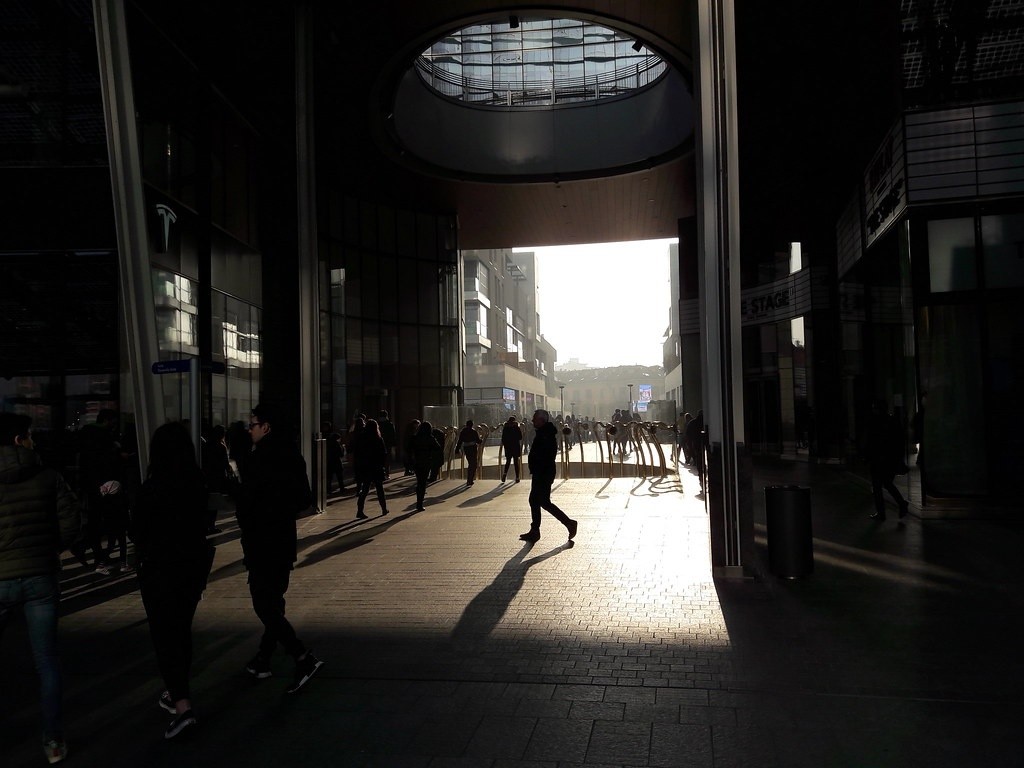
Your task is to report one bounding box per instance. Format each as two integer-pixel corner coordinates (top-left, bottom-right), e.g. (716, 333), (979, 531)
(247, 659), (272, 677)
(159, 690), (178, 715)
(42, 730), (66, 763)
(165, 709), (198, 737)
(288, 651), (325, 695)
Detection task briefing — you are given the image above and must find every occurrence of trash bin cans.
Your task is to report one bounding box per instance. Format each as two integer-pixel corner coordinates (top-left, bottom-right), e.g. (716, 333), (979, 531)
(764, 484), (815, 580)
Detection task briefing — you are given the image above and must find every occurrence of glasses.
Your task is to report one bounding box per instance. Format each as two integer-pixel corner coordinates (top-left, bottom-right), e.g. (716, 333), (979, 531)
(248, 421), (261, 429)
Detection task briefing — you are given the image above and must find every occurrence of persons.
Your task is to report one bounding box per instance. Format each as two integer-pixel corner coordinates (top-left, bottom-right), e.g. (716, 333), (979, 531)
(321, 420), (347, 498)
(218, 400), (325, 694)
(501, 416), (523, 483)
(403, 419), (445, 511)
(868, 399), (905, 525)
(346, 410), (396, 519)
(520, 410), (577, 541)
(0, 410), (81, 764)
(201, 417), (255, 535)
(78, 408), (138, 576)
(911, 411), (923, 471)
(455, 420), (483, 486)
(126, 418), (216, 740)
(676, 409), (704, 496)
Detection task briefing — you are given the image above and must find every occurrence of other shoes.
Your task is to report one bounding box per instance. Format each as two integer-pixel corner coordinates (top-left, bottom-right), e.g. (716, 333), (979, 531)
(120, 566), (133, 572)
(96, 568), (110, 575)
(416, 507), (425, 511)
(569, 520), (577, 539)
(382, 510), (389, 515)
(404, 472), (414, 475)
(502, 474), (506, 482)
(870, 512), (886, 522)
(520, 533), (540, 540)
(899, 501), (908, 519)
(516, 480), (520, 483)
(356, 512), (368, 518)
